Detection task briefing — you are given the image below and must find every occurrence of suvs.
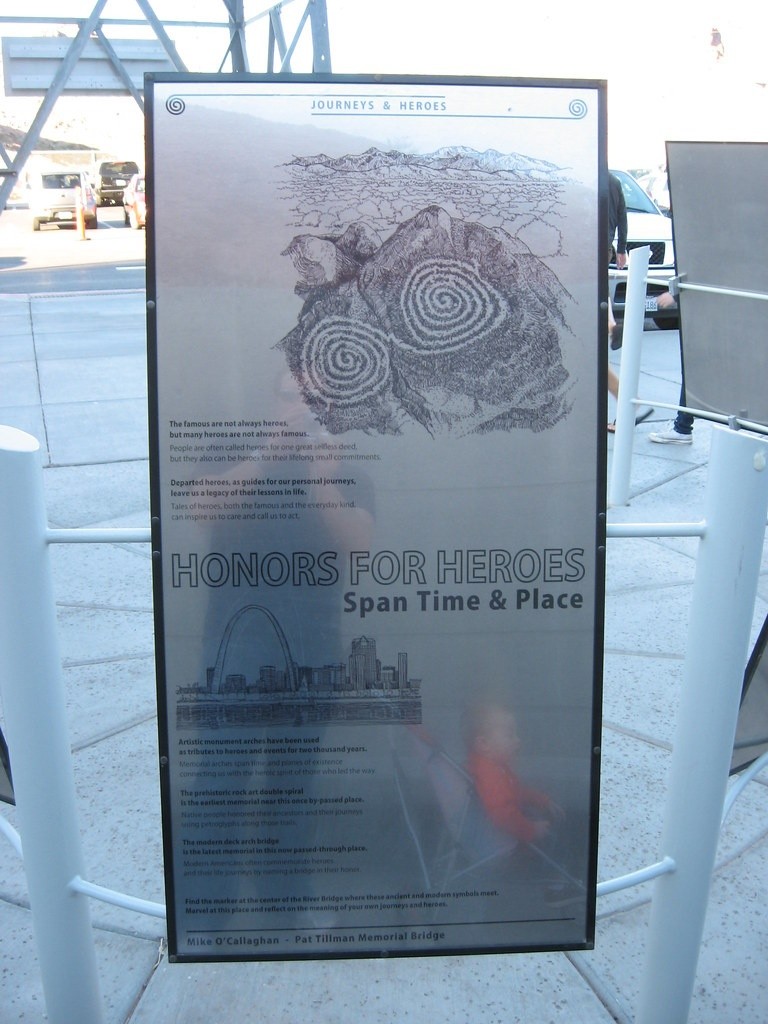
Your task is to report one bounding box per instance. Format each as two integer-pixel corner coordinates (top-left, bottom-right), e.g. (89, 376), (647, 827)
(97, 161), (141, 206)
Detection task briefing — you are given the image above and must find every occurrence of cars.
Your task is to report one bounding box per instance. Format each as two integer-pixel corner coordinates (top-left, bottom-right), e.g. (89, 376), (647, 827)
(120, 174), (147, 229)
(609, 169), (681, 331)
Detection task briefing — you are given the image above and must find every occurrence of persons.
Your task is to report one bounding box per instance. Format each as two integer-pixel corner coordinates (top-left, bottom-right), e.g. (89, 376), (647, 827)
(649, 291), (695, 443)
(607, 172), (654, 433)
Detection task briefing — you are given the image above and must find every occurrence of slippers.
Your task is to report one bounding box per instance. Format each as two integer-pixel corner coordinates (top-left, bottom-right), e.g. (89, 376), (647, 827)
(606, 406), (654, 433)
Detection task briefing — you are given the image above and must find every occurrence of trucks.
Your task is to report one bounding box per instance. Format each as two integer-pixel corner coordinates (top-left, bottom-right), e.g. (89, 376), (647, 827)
(25, 151), (101, 230)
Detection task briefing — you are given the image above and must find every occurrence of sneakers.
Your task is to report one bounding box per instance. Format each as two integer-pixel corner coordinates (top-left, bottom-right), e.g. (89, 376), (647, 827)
(650, 425), (693, 446)
